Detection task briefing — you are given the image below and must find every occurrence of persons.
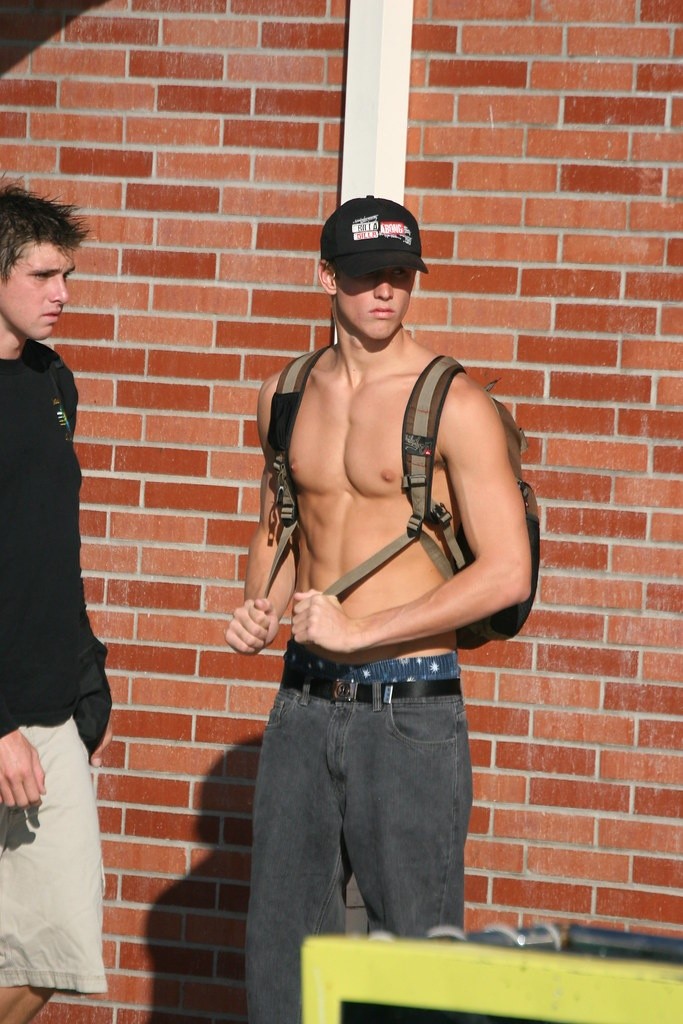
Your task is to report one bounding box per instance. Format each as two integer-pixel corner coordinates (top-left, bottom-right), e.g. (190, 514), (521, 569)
(0, 188), (115, 993)
(228, 196), (532, 1024)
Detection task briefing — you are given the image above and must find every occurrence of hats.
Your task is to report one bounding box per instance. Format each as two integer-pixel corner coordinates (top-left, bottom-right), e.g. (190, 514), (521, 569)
(320, 195), (429, 279)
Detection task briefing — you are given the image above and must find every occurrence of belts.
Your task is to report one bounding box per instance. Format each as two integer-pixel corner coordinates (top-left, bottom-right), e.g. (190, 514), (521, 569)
(281, 662), (461, 704)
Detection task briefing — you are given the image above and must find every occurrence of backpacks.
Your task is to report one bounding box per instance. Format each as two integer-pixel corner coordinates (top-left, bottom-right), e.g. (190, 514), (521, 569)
(254, 345), (541, 649)
